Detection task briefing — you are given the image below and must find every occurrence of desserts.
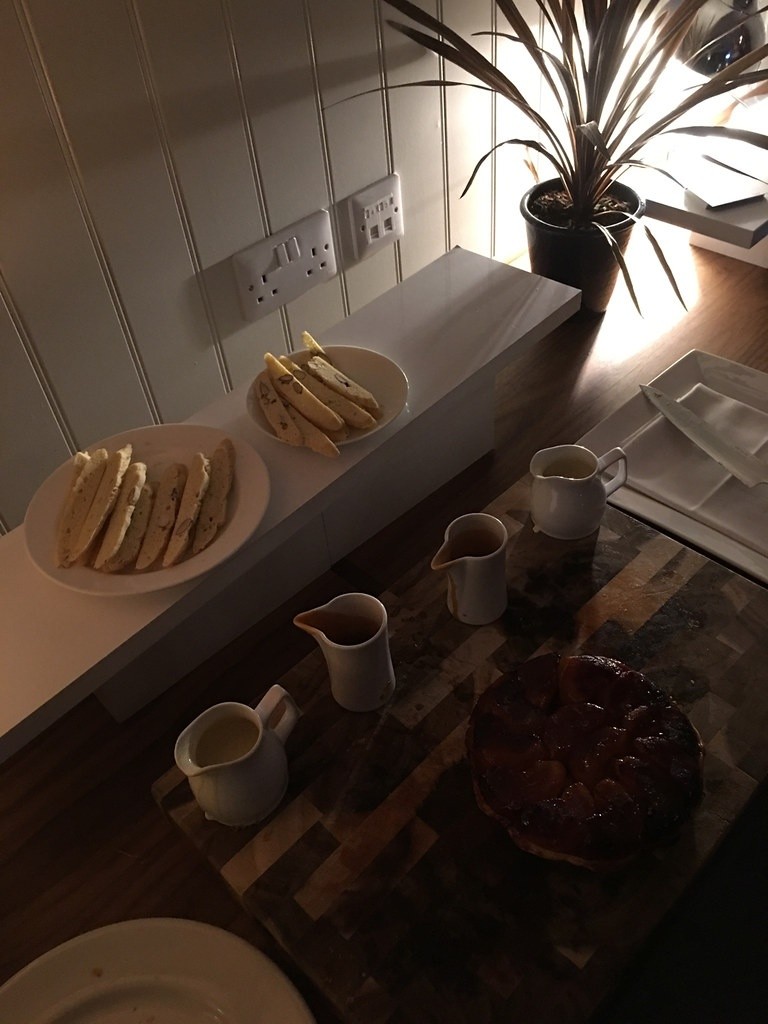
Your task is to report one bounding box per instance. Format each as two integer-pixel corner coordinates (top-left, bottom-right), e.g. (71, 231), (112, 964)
(462, 650), (707, 870)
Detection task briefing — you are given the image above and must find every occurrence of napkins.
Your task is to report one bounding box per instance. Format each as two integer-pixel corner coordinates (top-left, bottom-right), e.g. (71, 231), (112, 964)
(603, 382), (768, 558)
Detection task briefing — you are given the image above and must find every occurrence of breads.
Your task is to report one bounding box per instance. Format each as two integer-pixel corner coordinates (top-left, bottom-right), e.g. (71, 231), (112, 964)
(55, 438), (234, 571)
(254, 331), (384, 459)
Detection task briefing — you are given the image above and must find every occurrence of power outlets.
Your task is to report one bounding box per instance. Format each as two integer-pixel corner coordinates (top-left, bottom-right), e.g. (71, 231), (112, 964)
(226, 207), (338, 321)
(346, 173), (405, 263)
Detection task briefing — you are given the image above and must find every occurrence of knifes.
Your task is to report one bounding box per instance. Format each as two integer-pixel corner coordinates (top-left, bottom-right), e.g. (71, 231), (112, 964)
(639, 385), (768, 489)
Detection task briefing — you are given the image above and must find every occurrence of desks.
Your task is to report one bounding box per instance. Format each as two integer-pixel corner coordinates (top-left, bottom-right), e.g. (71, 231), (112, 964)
(149, 470), (768, 1024)
(0, 273), (581, 762)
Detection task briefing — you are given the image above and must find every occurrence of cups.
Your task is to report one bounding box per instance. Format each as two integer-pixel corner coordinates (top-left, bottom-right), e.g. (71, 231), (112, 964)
(531, 444), (628, 540)
(174, 683), (303, 826)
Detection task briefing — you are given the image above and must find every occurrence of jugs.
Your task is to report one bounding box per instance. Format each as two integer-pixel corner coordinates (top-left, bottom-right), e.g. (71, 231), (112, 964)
(294, 593), (396, 712)
(431, 513), (508, 626)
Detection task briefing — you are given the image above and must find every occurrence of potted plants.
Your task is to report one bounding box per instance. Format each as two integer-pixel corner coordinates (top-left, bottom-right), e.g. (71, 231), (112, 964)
(321, 0), (768, 318)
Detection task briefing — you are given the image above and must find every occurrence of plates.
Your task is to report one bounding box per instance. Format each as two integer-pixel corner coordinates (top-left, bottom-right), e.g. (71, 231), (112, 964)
(0, 918), (314, 1024)
(573, 351), (768, 584)
(472, 774), (635, 875)
(23, 422), (271, 596)
(245, 345), (409, 447)
